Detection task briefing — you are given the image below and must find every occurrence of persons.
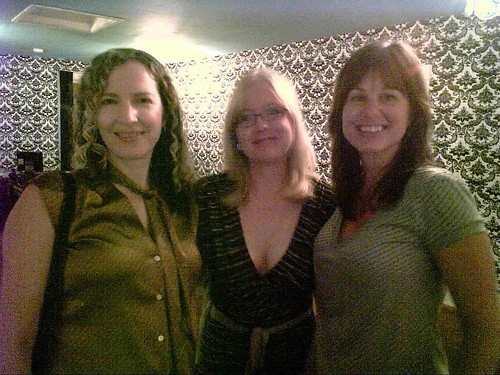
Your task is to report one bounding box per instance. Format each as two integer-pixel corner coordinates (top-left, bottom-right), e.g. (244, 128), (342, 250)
(190, 70), (337, 375)
(303, 43), (500, 375)
(0, 50), (202, 375)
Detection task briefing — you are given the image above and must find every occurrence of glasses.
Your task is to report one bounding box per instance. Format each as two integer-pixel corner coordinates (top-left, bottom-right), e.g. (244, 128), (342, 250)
(231, 107), (284, 120)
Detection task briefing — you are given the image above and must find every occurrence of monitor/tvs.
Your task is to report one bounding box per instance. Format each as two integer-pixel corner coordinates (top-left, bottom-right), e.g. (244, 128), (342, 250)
(17, 152), (43, 172)
